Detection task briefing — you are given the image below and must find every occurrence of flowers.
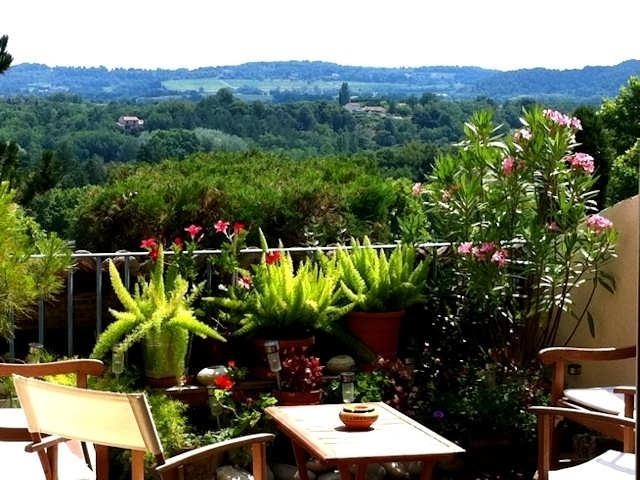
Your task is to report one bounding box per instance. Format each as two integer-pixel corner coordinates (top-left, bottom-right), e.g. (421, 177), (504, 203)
(397, 100), (618, 344)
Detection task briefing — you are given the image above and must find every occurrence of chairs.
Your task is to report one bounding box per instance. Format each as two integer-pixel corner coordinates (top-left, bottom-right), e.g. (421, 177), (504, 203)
(524, 343), (639, 479)
(2, 360), (275, 480)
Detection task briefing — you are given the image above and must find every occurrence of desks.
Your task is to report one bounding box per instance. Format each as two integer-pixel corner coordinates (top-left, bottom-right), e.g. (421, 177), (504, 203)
(260, 399), (467, 478)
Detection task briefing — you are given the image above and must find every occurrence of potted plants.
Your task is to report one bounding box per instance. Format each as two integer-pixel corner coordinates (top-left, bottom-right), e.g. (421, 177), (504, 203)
(433, 354), (555, 473)
